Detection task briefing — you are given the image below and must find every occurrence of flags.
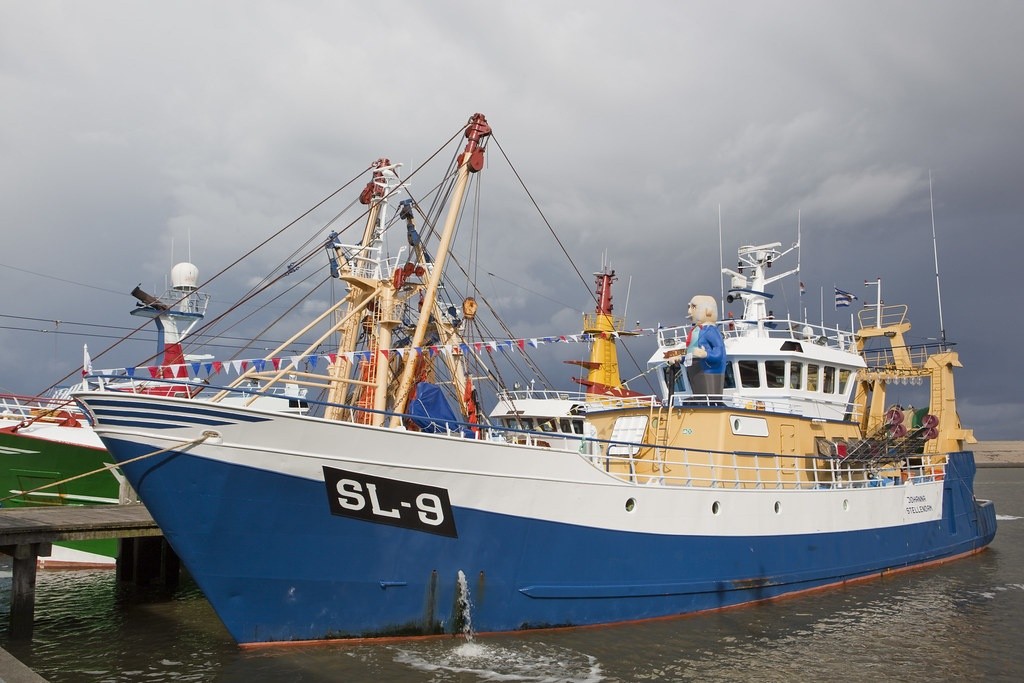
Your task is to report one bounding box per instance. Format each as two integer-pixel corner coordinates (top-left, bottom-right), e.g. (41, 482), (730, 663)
(834, 288), (857, 308)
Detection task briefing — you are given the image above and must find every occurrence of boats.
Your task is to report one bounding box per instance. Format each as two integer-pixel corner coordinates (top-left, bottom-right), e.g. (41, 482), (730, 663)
(1, 228), (311, 509)
(78, 110), (1002, 654)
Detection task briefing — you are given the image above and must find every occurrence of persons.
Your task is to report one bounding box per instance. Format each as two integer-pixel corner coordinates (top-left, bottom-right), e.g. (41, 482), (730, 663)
(666, 295), (727, 405)
